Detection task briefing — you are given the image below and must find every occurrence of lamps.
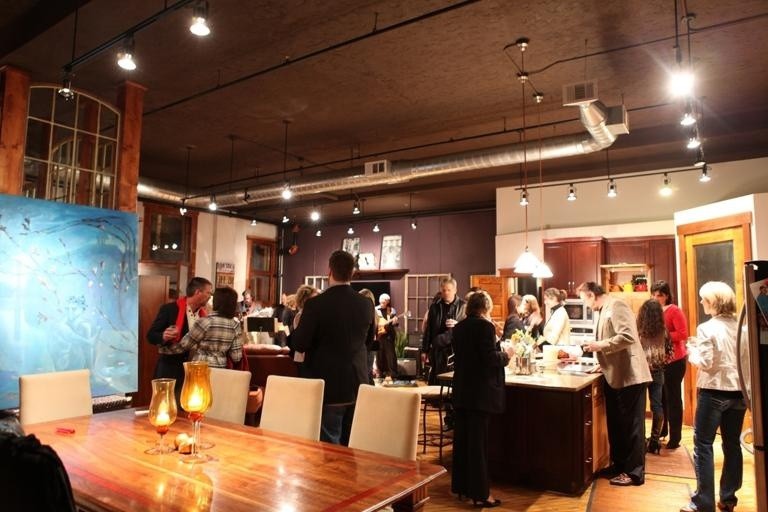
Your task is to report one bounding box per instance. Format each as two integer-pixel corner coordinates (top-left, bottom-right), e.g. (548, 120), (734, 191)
(515, 37), (544, 279)
(515, 163), (712, 207)
(670, 1), (713, 184)
(56, 1), (213, 103)
(178, 182), (361, 226)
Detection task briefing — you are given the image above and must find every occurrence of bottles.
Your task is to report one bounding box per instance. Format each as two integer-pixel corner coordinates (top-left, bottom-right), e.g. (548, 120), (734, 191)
(531, 356), (544, 374)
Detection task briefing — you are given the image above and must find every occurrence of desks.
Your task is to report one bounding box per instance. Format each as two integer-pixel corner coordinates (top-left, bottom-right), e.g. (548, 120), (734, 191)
(4, 402), (447, 512)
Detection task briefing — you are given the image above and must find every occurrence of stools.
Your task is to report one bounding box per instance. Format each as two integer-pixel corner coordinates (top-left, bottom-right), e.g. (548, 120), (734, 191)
(415, 385), (453, 460)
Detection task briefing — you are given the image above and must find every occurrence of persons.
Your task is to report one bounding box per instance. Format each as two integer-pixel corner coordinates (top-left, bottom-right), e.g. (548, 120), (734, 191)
(446, 289), (518, 508)
(678, 279), (753, 512)
(145, 275), (214, 413)
(238, 288), (264, 317)
(266, 282), (407, 381)
(164, 286), (245, 369)
(284, 249), (377, 447)
(420, 274), (687, 486)
(756, 284), (768, 320)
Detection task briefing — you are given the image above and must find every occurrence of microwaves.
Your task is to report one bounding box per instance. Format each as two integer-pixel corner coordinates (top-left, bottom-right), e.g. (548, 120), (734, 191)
(544, 299), (595, 326)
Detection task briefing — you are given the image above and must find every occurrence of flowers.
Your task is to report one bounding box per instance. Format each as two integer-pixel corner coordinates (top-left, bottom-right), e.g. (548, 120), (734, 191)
(511, 327), (536, 357)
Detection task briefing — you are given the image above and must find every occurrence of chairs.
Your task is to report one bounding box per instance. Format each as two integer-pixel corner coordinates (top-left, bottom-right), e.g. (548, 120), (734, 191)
(182, 365), (420, 470)
(16, 370), (94, 430)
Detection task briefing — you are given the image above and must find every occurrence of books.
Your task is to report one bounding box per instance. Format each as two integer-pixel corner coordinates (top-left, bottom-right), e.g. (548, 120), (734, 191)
(748, 275), (768, 326)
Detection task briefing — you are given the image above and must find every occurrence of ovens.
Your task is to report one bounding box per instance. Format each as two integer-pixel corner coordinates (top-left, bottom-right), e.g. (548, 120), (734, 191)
(541, 328), (595, 359)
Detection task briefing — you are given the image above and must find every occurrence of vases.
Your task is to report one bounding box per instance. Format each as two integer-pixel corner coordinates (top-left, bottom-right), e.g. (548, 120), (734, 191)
(514, 356), (532, 374)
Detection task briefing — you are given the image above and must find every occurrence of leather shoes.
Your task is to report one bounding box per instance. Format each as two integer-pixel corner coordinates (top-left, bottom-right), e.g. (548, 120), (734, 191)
(610, 472), (636, 485)
(604, 464), (619, 478)
(666, 439), (680, 448)
(680, 503), (696, 512)
(718, 502), (734, 512)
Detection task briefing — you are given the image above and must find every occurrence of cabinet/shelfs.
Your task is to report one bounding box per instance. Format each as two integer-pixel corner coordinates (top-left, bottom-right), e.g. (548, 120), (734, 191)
(490, 376), (609, 497)
(605, 240), (677, 304)
(544, 241), (606, 296)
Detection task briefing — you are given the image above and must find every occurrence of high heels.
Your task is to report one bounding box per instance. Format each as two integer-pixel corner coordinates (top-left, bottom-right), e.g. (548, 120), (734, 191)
(473, 497), (501, 506)
(659, 431), (668, 440)
(646, 438), (661, 454)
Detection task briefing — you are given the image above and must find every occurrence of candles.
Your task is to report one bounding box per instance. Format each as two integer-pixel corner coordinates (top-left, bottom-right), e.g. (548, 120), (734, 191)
(154, 382), (204, 426)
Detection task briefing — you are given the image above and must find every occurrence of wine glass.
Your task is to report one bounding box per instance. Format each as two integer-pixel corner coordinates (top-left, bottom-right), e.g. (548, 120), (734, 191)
(145, 377), (178, 455)
(180, 361), (215, 465)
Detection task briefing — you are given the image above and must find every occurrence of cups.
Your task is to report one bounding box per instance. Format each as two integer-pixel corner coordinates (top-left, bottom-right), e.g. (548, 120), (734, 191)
(624, 274), (647, 292)
(568, 281), (575, 295)
